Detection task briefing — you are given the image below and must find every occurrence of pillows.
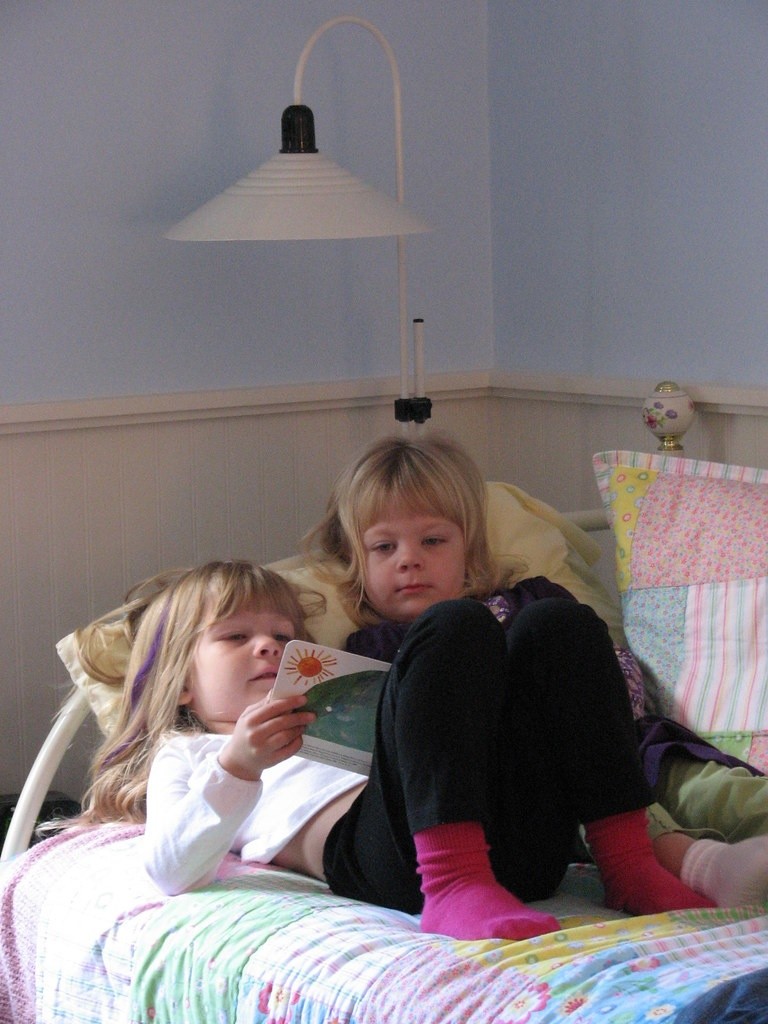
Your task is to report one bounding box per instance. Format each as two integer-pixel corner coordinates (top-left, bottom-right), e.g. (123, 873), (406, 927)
(592, 449), (768, 774)
(56, 550), (358, 740)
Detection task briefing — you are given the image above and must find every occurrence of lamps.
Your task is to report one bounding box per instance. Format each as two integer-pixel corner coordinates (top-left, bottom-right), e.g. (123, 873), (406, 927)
(163, 16), (432, 436)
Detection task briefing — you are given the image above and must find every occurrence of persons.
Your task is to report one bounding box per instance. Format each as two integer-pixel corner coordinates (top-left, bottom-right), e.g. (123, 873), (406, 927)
(311, 434), (767, 911)
(82, 559), (716, 941)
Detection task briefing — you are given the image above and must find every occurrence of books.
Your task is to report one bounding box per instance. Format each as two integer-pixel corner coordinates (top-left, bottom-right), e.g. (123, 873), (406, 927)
(265, 638), (393, 776)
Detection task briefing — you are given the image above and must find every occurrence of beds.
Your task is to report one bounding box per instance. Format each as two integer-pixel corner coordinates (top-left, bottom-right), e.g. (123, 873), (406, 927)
(0, 688), (768, 1024)
